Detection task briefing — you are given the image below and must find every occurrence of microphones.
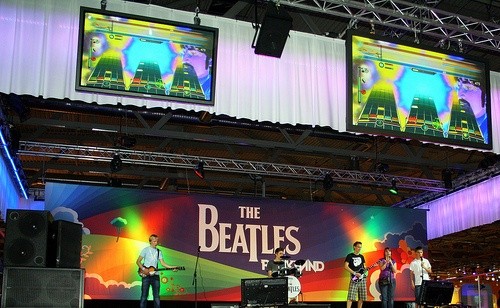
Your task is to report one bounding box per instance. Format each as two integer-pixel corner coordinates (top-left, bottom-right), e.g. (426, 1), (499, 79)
(388, 254), (391, 259)
(420, 256), (423, 262)
(197, 246), (201, 251)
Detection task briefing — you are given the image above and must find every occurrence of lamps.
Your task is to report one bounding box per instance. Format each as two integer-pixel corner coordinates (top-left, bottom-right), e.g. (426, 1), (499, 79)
(195, 161), (204, 179)
(110, 155), (122, 173)
(194, 7), (200, 25)
(390, 178), (398, 195)
(370, 23), (375, 34)
(101, 0), (107, 11)
(323, 174), (333, 190)
(251, 2), (293, 58)
(120, 135), (136, 148)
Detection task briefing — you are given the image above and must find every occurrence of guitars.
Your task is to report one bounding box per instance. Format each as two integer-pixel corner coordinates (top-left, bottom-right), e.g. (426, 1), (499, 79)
(351, 258), (384, 284)
(138, 266), (185, 278)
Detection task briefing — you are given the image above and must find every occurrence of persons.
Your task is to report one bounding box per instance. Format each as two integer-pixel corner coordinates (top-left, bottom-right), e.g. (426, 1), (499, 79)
(136, 234), (178, 308)
(378, 247), (397, 308)
(409, 246), (432, 308)
(266, 247), (288, 278)
(344, 242), (368, 308)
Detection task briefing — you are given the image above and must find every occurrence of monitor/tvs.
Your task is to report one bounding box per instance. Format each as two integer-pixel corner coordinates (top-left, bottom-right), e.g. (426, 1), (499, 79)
(75, 4), (218, 106)
(417, 280), (455, 305)
(344, 28), (494, 150)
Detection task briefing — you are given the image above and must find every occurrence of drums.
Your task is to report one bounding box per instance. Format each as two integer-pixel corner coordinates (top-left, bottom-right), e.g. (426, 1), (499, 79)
(282, 275), (301, 298)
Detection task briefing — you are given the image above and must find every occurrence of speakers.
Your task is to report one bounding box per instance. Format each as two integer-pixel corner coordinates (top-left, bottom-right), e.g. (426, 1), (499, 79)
(241, 277), (288, 308)
(253, 13), (294, 59)
(0, 209), (86, 308)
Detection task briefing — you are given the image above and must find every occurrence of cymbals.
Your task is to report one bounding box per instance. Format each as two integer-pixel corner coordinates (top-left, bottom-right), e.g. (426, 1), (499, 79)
(280, 256), (290, 258)
(295, 260), (305, 265)
(274, 262), (284, 264)
